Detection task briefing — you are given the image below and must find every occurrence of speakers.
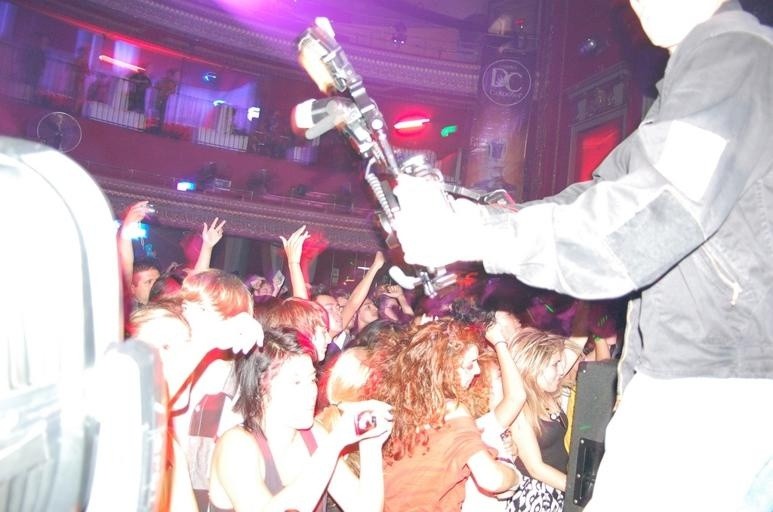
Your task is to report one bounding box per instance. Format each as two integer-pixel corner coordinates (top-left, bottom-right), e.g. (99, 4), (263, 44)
(563, 360), (618, 512)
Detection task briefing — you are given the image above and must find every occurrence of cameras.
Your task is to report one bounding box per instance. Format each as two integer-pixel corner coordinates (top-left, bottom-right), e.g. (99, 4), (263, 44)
(354, 410), (376, 435)
